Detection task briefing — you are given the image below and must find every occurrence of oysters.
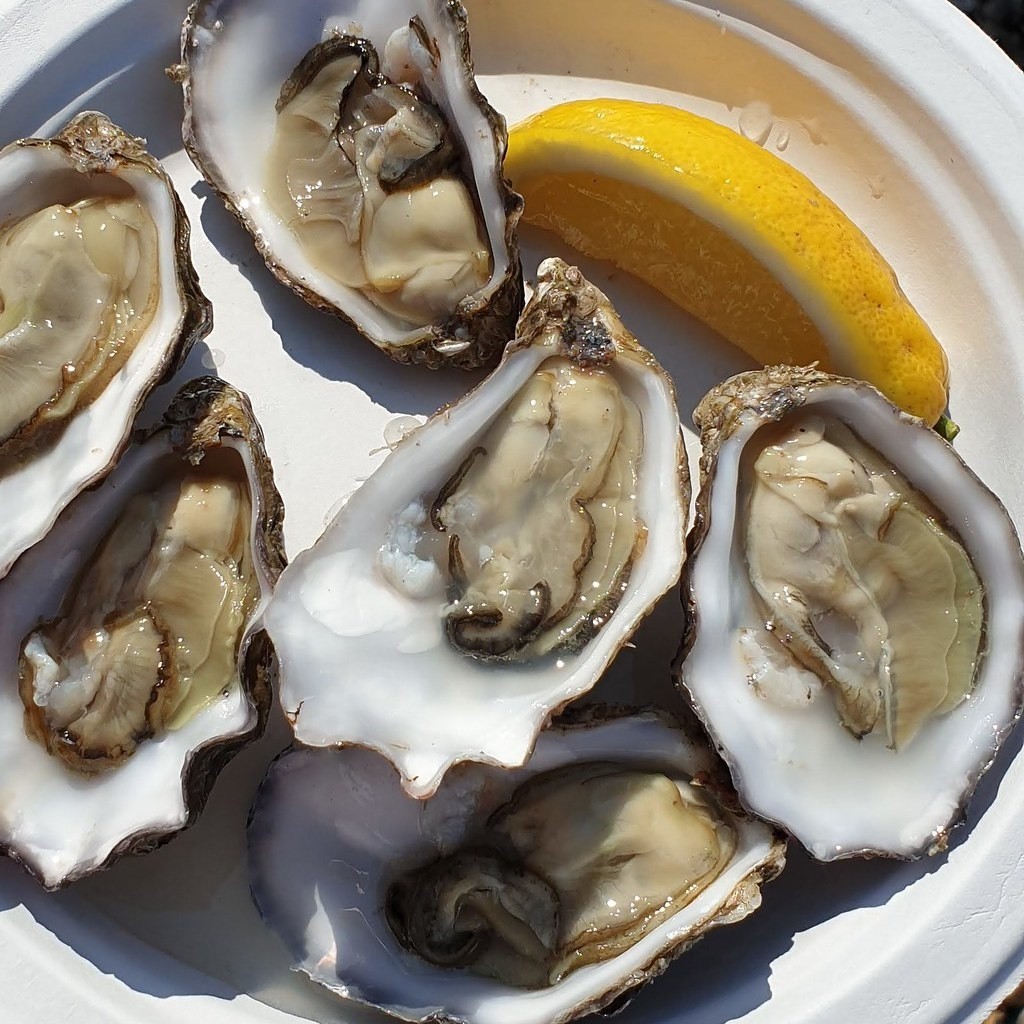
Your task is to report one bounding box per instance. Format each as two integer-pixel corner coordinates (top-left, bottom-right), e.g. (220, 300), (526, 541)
(0, 0), (1024, 1024)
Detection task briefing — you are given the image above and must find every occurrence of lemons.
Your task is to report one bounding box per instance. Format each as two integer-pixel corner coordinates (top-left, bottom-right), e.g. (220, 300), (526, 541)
(505, 97), (963, 439)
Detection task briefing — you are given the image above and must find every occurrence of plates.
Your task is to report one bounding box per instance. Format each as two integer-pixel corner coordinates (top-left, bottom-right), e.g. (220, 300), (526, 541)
(0, 1), (1024, 1024)
